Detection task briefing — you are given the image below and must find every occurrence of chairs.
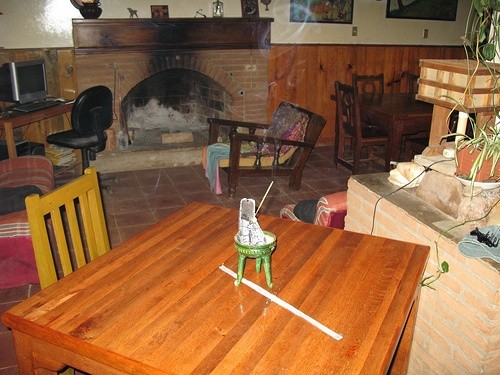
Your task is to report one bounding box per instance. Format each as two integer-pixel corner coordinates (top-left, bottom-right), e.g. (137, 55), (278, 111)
(0, 155), (57, 305)
(24, 167), (111, 290)
(334, 80), (389, 174)
(403, 110), (476, 162)
(46, 85), (115, 196)
(351, 73), (419, 97)
(206, 101), (328, 197)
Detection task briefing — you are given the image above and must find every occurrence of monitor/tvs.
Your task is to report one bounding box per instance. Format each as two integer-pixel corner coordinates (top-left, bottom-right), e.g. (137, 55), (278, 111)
(0, 59), (47, 107)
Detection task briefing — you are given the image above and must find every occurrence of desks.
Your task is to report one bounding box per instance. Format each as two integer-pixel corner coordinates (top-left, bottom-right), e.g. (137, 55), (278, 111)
(361, 93), (434, 170)
(0, 201), (430, 374)
(0, 95), (76, 159)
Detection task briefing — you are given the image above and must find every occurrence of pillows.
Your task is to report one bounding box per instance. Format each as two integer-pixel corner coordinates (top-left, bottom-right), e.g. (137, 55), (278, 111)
(293, 200), (319, 223)
(0, 185), (44, 215)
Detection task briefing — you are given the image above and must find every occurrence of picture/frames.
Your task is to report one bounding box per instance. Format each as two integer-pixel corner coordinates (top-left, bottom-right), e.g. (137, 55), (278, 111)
(290, 0), (354, 24)
(385, 0), (458, 21)
(241, 0), (260, 19)
(151, 5), (169, 19)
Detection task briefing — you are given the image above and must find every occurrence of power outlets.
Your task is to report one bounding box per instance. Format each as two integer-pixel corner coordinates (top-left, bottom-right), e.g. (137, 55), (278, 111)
(352, 27), (358, 36)
(423, 29), (428, 39)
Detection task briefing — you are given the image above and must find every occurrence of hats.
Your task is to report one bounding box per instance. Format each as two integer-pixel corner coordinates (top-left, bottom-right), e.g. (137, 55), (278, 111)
(457, 225), (500, 264)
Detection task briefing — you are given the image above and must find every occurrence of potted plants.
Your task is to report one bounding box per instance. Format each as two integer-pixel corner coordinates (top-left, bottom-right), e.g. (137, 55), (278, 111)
(438, 115), (500, 190)
(446, 0), (500, 134)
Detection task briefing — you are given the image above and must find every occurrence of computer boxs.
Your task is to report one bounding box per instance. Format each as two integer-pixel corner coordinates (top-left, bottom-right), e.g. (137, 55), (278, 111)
(0, 140), (30, 160)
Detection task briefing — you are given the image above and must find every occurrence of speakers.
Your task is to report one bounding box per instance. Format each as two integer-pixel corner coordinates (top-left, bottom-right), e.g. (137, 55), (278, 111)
(29, 142), (44, 155)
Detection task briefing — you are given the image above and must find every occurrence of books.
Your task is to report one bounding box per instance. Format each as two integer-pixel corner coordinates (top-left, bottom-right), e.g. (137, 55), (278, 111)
(45, 144), (77, 166)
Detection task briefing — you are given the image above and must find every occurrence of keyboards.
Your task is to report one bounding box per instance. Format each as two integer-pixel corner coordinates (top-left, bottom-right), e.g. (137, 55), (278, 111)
(12, 100), (61, 113)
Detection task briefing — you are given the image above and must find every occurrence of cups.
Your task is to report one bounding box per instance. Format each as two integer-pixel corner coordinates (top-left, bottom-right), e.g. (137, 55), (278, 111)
(213, 3), (224, 17)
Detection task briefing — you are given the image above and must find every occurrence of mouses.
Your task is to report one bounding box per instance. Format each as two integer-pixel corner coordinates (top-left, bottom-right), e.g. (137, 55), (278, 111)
(54, 98), (66, 102)
